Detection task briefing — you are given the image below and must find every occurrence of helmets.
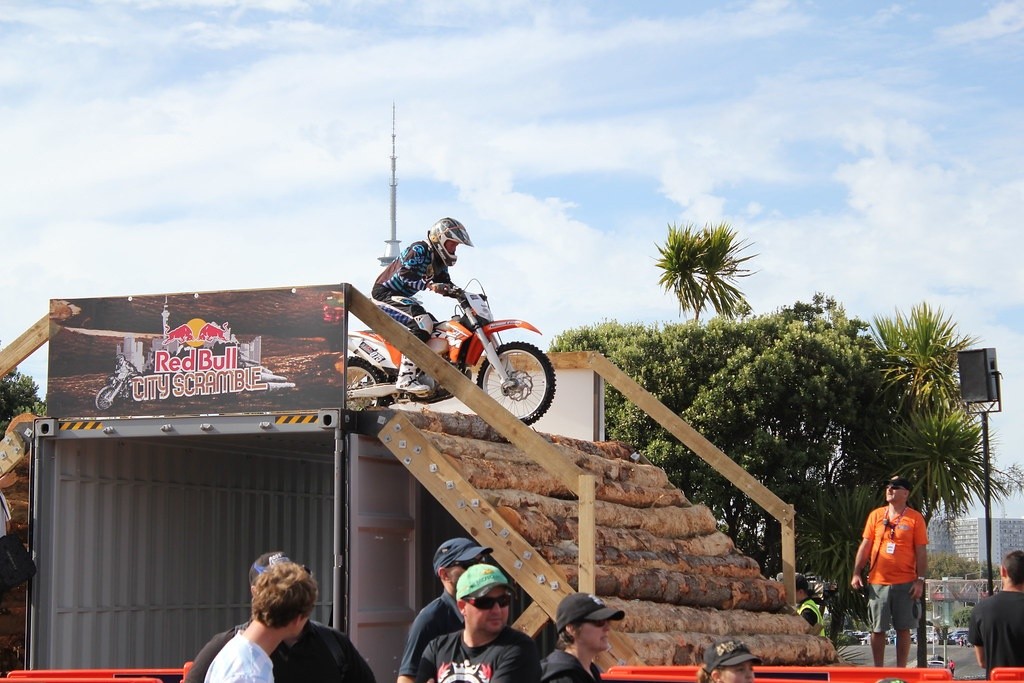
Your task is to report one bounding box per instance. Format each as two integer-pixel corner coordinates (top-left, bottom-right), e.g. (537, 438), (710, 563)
(429, 218), (474, 267)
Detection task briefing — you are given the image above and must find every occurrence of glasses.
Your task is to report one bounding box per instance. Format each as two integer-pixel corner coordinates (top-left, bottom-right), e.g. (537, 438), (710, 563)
(584, 619), (606, 627)
(885, 485), (903, 489)
(449, 557), (486, 570)
(463, 593), (511, 609)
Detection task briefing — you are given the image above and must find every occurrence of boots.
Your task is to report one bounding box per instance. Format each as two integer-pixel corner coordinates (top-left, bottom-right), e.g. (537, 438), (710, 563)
(395, 354), (430, 394)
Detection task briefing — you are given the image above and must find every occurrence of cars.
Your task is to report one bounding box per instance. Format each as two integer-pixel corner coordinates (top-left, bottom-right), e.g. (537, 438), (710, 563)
(852, 626), (970, 646)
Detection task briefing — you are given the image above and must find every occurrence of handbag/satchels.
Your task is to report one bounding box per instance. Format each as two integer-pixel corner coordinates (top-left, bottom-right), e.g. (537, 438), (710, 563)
(0, 533), (36, 591)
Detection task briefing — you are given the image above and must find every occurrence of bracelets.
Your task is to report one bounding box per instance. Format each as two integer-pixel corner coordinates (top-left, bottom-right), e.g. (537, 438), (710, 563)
(917, 576), (926, 580)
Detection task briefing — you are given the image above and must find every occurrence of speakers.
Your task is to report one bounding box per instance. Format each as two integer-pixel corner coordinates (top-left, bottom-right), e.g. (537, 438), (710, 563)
(957, 348), (998, 403)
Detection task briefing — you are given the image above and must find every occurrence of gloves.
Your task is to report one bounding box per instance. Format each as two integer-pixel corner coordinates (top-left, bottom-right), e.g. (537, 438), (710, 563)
(427, 281), (444, 292)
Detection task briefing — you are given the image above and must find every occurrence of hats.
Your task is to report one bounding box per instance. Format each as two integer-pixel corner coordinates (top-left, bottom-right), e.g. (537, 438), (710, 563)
(455, 563), (508, 599)
(881, 475), (913, 491)
(776, 572), (808, 590)
(433, 537), (494, 578)
(249, 552), (311, 588)
(704, 638), (762, 672)
(556, 592), (626, 633)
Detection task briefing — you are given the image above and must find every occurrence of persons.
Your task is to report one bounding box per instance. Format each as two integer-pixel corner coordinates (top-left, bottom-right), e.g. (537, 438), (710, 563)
(541, 593), (626, 683)
(960, 634), (970, 647)
(182, 552), (376, 683)
(396, 538), (543, 683)
(371, 217), (474, 391)
(0, 490), (14, 614)
(948, 659), (957, 676)
(795, 574), (825, 637)
(851, 476), (929, 668)
(698, 638), (762, 683)
(968, 550), (1024, 680)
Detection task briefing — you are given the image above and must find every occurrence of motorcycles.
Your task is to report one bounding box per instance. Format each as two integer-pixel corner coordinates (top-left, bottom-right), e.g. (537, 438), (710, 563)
(347, 274), (556, 420)
